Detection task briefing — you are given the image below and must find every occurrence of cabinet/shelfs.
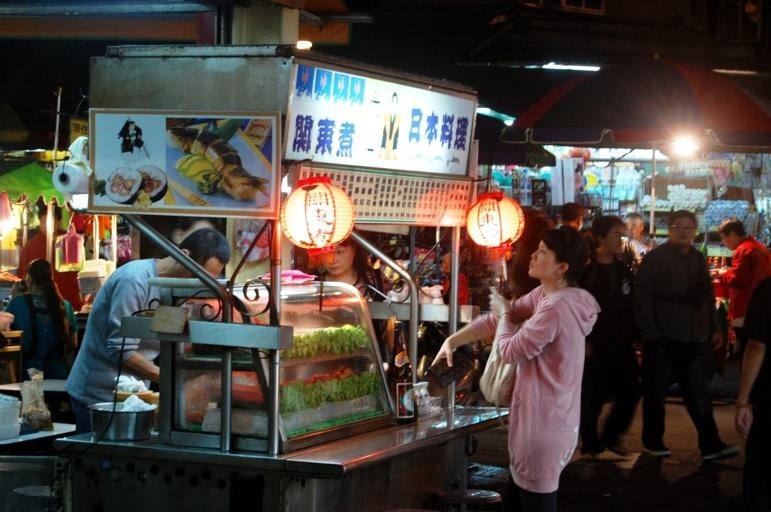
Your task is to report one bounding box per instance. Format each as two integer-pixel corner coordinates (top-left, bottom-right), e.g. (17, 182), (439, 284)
(158, 282), (397, 455)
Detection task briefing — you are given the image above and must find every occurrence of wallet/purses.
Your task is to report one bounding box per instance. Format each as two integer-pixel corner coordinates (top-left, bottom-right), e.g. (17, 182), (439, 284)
(427, 350), (474, 388)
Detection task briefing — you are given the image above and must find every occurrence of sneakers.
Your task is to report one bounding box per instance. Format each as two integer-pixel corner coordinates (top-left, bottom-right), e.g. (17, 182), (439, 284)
(580, 444), (670, 460)
(702, 443), (740, 459)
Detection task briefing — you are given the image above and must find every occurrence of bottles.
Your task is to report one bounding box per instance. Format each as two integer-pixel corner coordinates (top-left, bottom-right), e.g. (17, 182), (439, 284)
(413, 382), (431, 415)
(705, 199), (751, 227)
(639, 184), (707, 210)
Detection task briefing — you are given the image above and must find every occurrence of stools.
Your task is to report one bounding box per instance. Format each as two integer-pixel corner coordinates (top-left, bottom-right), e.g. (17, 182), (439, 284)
(437, 489), (501, 512)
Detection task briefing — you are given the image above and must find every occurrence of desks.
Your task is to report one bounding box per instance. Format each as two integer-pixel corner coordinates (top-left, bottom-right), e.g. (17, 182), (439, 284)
(52, 136), (93, 194)
(0, 330), (77, 445)
(56, 405), (510, 512)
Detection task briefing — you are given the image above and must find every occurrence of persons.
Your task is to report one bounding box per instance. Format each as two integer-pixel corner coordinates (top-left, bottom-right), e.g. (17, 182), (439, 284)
(15, 202), (85, 314)
(317, 239), (408, 367)
(63, 226), (231, 432)
(5, 260), (74, 380)
(117, 119), (144, 153)
(60, 297), (79, 348)
(426, 203), (771, 512)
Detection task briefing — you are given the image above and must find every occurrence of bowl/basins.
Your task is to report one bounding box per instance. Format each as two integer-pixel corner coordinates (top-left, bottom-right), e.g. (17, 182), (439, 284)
(87, 402), (156, 440)
(0, 394), (22, 440)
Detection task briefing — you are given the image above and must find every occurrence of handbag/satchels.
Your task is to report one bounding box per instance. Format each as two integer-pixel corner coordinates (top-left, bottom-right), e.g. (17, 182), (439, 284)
(479, 321), (518, 406)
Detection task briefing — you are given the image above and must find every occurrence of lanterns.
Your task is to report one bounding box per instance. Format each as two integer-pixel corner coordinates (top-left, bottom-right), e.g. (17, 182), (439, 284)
(464, 191), (527, 264)
(281, 174), (358, 268)
